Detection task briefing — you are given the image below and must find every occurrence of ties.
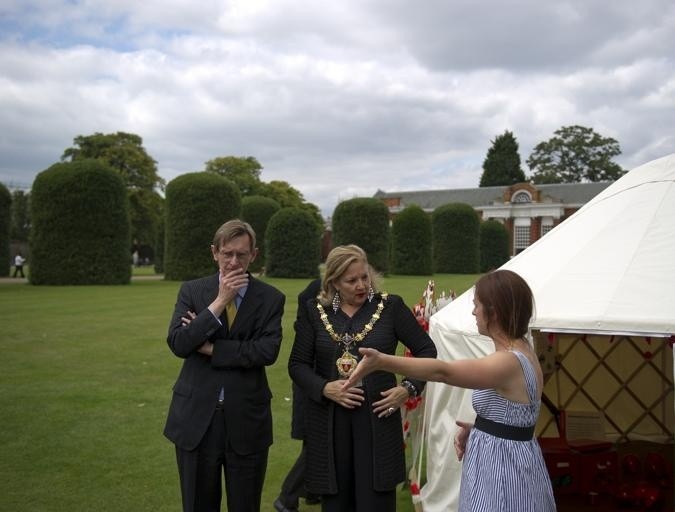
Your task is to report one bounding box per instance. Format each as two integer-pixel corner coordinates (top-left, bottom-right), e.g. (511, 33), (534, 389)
(225, 299), (238, 331)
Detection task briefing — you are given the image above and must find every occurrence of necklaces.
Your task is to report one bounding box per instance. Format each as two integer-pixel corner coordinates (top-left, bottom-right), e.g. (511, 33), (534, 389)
(313, 291), (390, 381)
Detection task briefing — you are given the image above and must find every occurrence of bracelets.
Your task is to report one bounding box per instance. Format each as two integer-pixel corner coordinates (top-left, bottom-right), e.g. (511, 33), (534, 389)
(400, 380), (417, 401)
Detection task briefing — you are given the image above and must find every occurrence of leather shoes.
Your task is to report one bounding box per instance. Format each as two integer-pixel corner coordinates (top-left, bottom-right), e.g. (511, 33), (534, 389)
(272, 498), (299, 512)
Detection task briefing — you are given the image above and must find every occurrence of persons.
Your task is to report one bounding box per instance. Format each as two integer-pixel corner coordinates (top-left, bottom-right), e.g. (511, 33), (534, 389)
(132, 247), (151, 268)
(163, 218), (286, 512)
(339, 269), (557, 512)
(288, 244), (438, 512)
(12, 252), (26, 278)
(274, 276), (331, 512)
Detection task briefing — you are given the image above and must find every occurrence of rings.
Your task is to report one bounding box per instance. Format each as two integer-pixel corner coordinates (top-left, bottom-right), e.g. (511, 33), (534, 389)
(388, 407), (394, 414)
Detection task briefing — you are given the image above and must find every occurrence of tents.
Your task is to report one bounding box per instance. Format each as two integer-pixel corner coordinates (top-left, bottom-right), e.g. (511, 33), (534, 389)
(417, 151), (675, 511)
